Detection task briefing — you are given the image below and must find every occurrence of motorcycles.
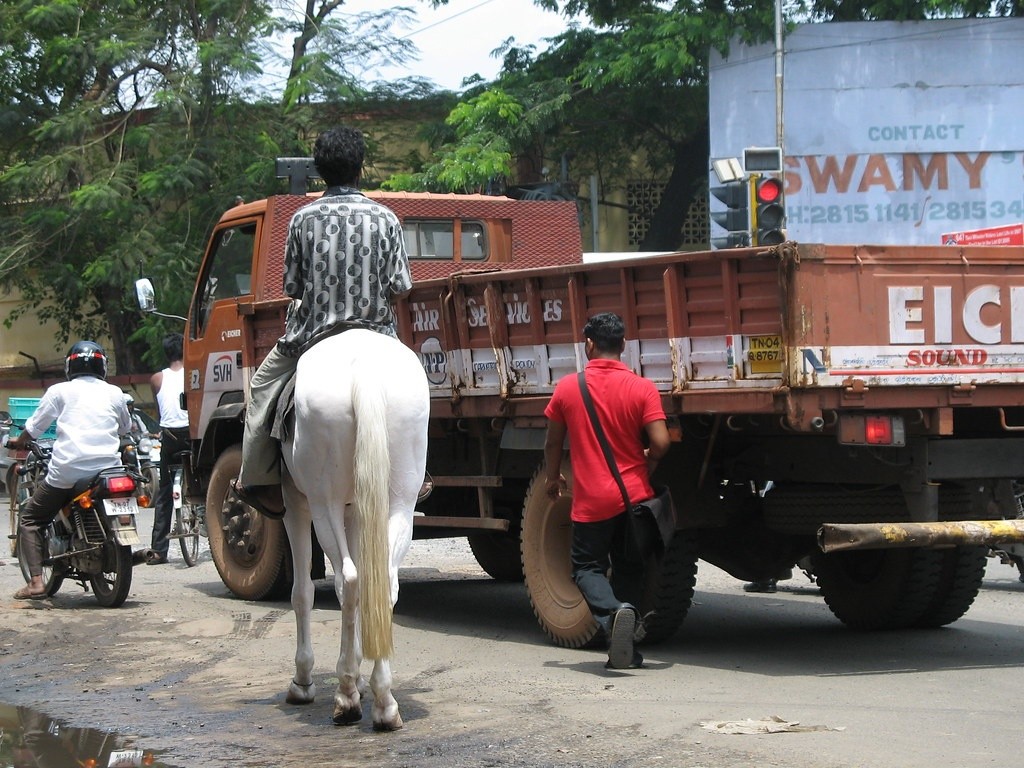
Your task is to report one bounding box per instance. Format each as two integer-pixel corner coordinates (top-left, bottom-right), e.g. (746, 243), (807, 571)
(0, 411), (150, 608)
(123, 431), (162, 508)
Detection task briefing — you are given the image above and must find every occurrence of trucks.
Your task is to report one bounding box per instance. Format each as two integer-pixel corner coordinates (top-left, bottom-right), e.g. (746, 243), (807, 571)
(135, 157), (1024, 649)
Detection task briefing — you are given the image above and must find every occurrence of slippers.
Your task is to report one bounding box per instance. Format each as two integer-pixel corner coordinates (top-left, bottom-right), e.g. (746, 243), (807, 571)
(13, 587), (48, 600)
(229, 478), (287, 519)
(416, 482), (433, 504)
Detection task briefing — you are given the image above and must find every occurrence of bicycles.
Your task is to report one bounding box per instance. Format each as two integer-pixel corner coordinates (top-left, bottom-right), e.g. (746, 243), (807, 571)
(165, 449), (208, 567)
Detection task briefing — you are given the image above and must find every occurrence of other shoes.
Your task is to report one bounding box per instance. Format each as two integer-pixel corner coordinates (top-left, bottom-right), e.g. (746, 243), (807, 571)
(608, 609), (635, 668)
(634, 605), (658, 644)
(146, 551), (168, 565)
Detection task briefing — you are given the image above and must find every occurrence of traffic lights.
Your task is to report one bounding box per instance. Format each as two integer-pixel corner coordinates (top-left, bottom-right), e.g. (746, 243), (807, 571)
(710, 185), (749, 249)
(756, 177), (786, 247)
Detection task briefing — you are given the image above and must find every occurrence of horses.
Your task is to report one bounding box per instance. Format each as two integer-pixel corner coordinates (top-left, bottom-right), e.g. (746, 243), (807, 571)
(278, 328), (431, 734)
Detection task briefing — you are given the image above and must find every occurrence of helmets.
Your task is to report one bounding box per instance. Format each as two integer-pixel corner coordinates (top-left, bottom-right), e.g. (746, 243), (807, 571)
(63, 340), (107, 380)
(123, 393), (134, 404)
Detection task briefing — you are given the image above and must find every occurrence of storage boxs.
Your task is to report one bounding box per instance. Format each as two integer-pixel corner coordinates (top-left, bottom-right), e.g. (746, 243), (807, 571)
(7, 396), (59, 460)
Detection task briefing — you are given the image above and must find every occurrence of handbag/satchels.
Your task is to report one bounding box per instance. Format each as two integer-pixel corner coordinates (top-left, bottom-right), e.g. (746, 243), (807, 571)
(624, 490), (679, 567)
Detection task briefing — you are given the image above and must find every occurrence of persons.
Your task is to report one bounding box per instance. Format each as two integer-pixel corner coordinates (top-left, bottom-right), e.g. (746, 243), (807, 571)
(230, 129), (432, 519)
(544, 312), (671, 669)
(7, 332), (207, 601)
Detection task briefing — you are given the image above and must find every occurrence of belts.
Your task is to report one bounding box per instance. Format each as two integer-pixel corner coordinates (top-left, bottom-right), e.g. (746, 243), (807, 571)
(162, 426), (189, 441)
